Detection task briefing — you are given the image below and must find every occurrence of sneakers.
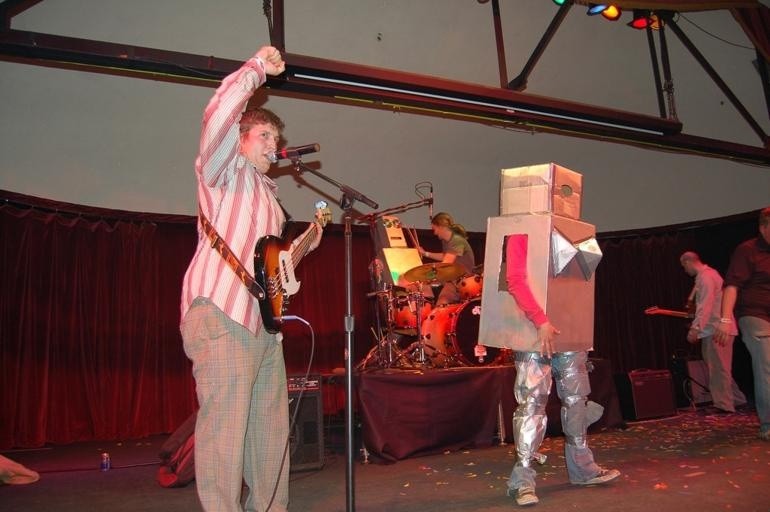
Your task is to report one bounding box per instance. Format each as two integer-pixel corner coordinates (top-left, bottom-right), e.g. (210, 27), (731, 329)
(512, 484), (539, 506)
(570, 467), (621, 485)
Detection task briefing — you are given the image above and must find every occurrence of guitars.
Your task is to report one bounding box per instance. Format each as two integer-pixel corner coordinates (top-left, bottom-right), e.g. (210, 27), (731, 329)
(644, 304), (696, 329)
(253, 199), (332, 334)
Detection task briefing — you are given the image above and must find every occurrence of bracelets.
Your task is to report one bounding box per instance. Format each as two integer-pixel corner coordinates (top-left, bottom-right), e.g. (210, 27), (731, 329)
(424, 251), (429, 257)
(694, 326), (699, 330)
(249, 56), (266, 73)
(718, 318), (732, 323)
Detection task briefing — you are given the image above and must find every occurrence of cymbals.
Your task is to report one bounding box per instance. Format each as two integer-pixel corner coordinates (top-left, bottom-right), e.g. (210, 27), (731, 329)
(404, 263), (465, 284)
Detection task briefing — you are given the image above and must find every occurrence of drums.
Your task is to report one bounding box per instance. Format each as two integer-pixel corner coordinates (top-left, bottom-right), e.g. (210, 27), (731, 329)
(420, 297), (501, 367)
(393, 291), (431, 337)
(456, 272), (483, 302)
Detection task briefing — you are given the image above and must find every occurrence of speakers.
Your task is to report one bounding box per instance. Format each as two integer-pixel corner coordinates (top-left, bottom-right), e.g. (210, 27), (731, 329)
(285, 371), (325, 474)
(616, 367), (677, 422)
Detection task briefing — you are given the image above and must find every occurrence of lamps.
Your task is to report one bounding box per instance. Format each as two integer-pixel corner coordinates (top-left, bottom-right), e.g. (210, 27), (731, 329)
(555, 0), (667, 28)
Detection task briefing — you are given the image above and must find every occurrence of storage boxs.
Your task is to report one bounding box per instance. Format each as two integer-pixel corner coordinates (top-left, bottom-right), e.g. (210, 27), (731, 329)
(500, 163), (582, 219)
(478, 214), (595, 353)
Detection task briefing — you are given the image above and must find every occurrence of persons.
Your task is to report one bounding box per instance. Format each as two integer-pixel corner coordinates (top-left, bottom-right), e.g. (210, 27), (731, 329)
(503, 234), (624, 505)
(413, 211), (476, 309)
(678, 250), (751, 416)
(711, 207), (769, 443)
(177, 45), (324, 512)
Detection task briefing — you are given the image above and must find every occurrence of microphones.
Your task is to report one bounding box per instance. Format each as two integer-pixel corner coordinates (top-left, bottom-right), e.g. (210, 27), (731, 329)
(266, 142), (320, 163)
(429, 184), (434, 222)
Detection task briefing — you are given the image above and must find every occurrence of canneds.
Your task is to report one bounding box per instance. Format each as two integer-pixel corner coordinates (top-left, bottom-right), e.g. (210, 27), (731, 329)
(101, 452), (111, 472)
